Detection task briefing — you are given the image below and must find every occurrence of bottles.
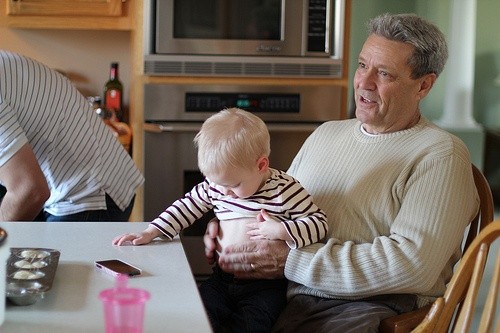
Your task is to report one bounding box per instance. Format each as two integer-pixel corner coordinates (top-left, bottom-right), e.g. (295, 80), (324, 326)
(102, 62), (124, 120)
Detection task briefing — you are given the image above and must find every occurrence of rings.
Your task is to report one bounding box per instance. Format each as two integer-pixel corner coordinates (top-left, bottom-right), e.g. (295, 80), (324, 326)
(250, 262), (256, 273)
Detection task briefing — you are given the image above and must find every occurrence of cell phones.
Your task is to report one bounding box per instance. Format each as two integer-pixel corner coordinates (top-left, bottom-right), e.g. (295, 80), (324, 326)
(95, 260), (142, 278)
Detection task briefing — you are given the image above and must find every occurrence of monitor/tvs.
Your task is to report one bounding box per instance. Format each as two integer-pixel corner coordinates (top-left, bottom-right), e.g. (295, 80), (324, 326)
(154, 0), (306, 56)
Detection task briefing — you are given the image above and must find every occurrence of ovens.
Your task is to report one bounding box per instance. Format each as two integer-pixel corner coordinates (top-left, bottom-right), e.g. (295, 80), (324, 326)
(144, 80), (341, 275)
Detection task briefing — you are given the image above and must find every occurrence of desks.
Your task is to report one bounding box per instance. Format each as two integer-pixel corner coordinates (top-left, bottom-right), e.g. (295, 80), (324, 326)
(410, 163), (500, 333)
(0, 221), (213, 333)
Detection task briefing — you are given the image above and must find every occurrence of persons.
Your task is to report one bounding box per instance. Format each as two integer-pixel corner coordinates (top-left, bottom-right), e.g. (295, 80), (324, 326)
(204, 11), (479, 333)
(0, 49), (145, 222)
(111, 108), (330, 333)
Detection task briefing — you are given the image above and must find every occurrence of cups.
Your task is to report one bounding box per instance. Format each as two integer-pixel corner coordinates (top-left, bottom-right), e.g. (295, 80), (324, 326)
(100, 287), (149, 333)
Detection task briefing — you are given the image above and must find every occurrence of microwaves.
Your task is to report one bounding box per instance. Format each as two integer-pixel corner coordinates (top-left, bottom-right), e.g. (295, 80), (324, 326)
(153, 0), (333, 56)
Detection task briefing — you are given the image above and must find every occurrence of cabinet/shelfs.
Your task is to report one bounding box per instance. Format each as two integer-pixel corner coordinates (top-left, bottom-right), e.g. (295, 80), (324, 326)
(0, 0), (132, 31)
